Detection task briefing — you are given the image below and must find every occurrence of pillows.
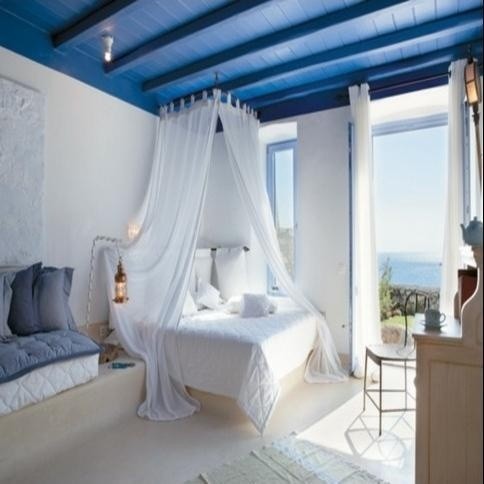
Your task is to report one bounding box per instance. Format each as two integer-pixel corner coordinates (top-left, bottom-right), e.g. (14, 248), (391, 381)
(1, 270), (17, 341)
(181, 279), (282, 318)
(32, 268), (78, 333)
(8, 260), (44, 337)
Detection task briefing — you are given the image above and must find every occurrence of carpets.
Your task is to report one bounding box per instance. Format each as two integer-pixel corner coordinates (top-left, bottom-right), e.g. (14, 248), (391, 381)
(181, 430), (389, 484)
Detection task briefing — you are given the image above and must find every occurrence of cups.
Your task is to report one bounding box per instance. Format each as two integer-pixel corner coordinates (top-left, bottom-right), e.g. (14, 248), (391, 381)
(421, 309), (447, 328)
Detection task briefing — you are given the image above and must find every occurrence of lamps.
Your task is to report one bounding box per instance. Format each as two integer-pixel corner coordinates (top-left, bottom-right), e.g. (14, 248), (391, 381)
(462, 43), (482, 106)
(112, 256), (130, 303)
(102, 36), (114, 63)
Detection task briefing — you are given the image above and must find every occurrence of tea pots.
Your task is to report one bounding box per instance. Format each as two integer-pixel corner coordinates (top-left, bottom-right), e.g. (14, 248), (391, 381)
(459, 216), (484, 245)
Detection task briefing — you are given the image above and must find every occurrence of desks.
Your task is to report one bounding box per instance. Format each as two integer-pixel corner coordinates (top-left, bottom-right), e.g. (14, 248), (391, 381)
(411, 245), (484, 484)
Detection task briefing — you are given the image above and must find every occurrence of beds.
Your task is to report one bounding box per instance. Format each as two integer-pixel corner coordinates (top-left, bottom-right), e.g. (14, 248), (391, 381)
(95, 235), (323, 399)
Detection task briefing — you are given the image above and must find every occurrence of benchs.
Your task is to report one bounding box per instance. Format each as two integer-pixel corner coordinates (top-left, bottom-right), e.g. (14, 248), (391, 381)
(0, 330), (104, 417)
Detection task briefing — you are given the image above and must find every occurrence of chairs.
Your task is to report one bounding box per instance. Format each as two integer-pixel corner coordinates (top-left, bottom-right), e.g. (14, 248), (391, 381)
(361, 291), (432, 437)
